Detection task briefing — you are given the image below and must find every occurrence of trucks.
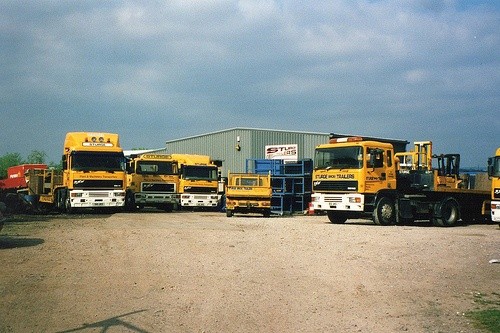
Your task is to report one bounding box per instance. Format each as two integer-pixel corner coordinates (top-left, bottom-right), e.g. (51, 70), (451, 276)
(1, 164), (48, 216)
(125, 153), (179, 213)
(225, 170), (272, 218)
(488, 147), (500, 227)
(54, 131), (125, 214)
(308, 136), (489, 227)
(172, 154), (222, 211)
